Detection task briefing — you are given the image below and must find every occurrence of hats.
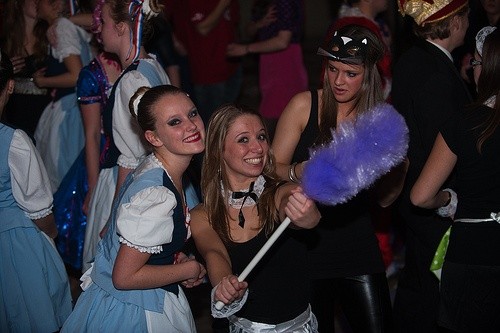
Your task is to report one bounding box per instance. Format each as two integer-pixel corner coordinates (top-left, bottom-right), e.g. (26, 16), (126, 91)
(398, 0), (468, 25)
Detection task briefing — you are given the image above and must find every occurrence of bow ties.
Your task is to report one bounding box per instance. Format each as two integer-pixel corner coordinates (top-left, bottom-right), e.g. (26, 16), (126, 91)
(231, 180), (260, 229)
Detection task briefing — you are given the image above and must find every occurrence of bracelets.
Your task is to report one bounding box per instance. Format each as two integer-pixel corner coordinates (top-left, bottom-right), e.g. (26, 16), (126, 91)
(288, 162), (303, 185)
(437, 188), (458, 218)
(210, 280), (249, 319)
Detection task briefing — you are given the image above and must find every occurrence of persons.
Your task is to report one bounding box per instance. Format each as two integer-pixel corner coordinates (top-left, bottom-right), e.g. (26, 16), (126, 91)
(189, 105), (322, 333)
(322, 0), (500, 278)
(394, 0), (474, 333)
(59, 85), (207, 333)
(271, 23), (409, 333)
(82, 0), (200, 273)
(0, 0), (308, 307)
(0, 50), (74, 333)
(410, 26), (500, 333)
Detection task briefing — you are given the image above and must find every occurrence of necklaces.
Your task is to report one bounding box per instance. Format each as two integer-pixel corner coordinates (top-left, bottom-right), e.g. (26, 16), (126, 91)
(220, 175), (266, 229)
